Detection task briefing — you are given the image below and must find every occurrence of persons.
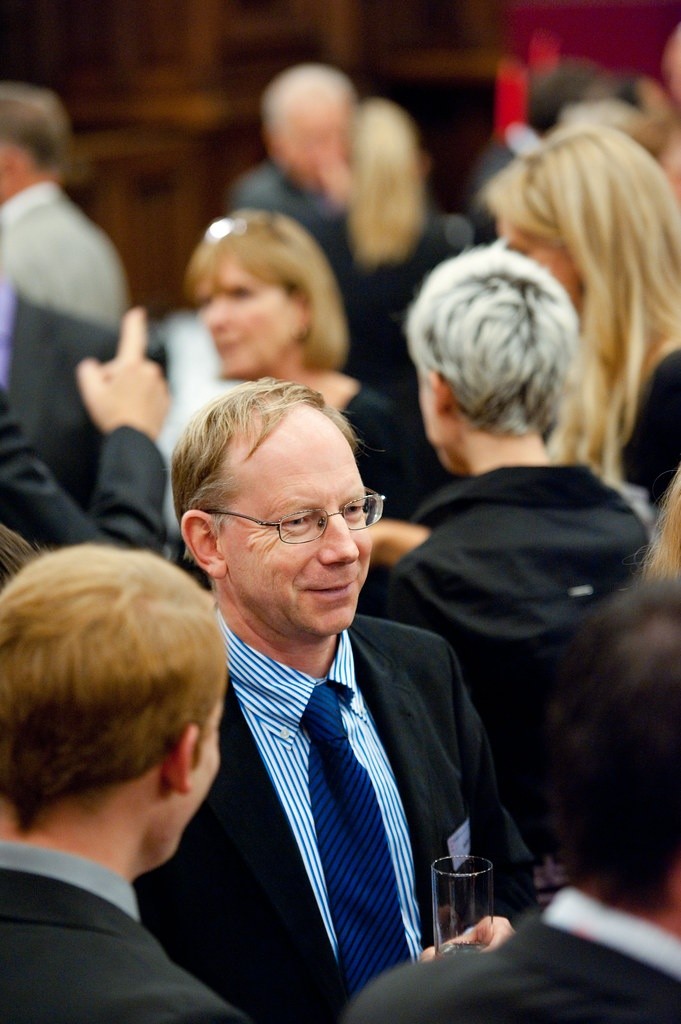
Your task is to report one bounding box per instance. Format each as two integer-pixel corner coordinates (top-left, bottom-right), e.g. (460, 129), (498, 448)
(1, 543), (244, 1022)
(305, 95), (478, 533)
(0, 297), (178, 561)
(1, 81), (134, 340)
(0, 519), (49, 597)
(127, 376), (541, 1022)
(480, 115), (681, 516)
(233, 63), (366, 228)
(186, 205), (452, 616)
(348, 570), (681, 1024)
(472, 67), (634, 255)
(351, 244), (650, 862)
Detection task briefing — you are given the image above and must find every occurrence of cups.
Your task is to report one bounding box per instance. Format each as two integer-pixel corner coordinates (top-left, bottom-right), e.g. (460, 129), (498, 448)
(429, 853), (496, 963)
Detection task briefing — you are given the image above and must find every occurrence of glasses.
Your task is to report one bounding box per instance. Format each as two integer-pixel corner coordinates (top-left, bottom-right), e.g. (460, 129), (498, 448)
(205, 486), (385, 545)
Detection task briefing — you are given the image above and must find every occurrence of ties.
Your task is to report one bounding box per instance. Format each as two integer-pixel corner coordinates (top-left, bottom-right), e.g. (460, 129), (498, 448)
(300, 685), (413, 990)
